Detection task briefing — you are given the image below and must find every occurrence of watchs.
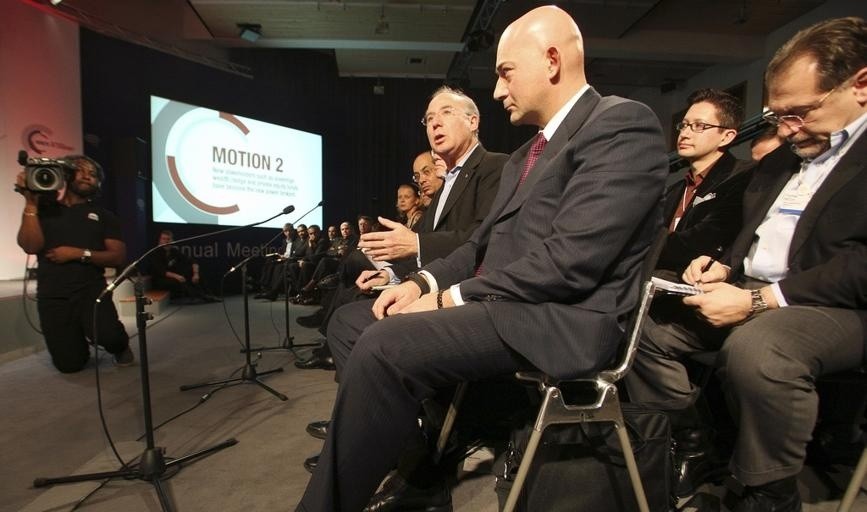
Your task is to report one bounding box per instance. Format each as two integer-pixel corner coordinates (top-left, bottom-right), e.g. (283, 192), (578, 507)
(81, 248), (92, 263)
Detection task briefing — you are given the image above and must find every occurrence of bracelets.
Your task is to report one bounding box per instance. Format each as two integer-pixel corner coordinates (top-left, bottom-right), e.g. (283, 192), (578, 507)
(23, 212), (39, 216)
(192, 272), (199, 273)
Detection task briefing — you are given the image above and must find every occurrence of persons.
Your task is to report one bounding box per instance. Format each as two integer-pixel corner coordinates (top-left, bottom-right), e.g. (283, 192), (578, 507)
(141, 232), (200, 306)
(16, 154), (134, 374)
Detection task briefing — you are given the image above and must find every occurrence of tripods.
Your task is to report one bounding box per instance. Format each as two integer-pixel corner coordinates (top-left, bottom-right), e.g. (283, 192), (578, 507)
(240, 268), (322, 361)
(34, 296), (238, 512)
(180, 272), (287, 401)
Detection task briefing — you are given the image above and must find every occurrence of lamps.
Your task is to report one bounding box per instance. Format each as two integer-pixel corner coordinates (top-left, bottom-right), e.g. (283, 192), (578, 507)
(373, 76), (385, 96)
(234, 22), (262, 43)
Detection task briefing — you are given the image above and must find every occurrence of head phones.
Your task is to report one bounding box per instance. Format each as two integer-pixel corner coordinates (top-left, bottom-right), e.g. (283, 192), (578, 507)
(63, 155), (106, 193)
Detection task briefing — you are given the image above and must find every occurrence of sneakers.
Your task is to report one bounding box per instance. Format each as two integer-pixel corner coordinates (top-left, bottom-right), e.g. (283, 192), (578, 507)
(113, 346), (134, 367)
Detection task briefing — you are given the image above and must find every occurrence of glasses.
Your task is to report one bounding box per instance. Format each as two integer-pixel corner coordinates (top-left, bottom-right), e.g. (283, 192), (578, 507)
(413, 168), (436, 182)
(763, 90), (836, 127)
(676, 122), (728, 131)
(420, 108), (471, 126)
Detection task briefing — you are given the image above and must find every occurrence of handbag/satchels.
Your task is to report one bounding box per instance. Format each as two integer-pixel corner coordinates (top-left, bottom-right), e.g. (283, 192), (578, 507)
(497, 402), (672, 512)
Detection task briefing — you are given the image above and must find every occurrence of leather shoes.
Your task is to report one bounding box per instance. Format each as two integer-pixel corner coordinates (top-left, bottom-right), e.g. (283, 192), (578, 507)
(294, 353), (336, 370)
(363, 474), (454, 512)
(304, 421), (330, 440)
(674, 443), (802, 512)
(297, 308), (327, 327)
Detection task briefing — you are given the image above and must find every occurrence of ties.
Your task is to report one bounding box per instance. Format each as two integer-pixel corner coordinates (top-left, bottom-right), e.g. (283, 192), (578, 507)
(515, 133), (547, 191)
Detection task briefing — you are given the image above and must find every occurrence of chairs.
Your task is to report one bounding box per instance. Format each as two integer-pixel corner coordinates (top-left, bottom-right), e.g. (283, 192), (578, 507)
(483, 220), (668, 512)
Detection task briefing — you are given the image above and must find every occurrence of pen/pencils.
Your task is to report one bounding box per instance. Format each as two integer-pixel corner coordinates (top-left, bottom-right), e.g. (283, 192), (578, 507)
(694, 245), (723, 289)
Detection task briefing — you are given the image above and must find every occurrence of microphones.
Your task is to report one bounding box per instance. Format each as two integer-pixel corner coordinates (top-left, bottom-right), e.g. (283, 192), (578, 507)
(95, 205), (295, 302)
(223, 200), (328, 279)
